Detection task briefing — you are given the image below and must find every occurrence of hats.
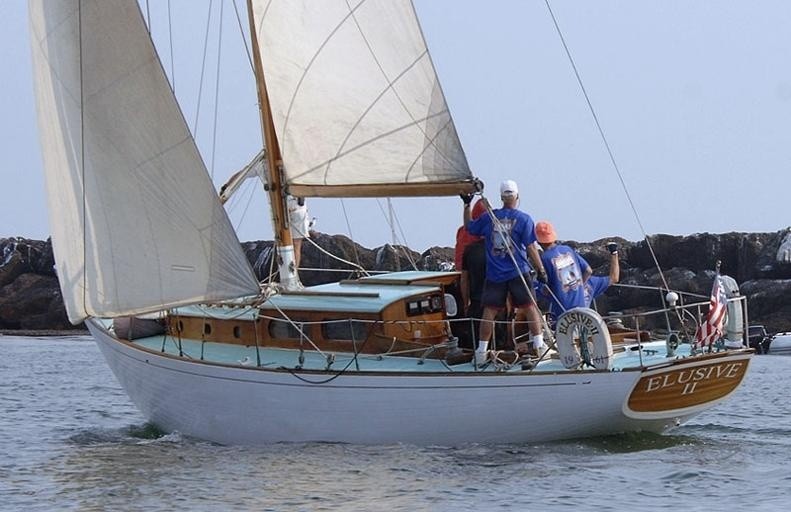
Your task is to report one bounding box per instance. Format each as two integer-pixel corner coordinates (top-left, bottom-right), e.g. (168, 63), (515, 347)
(500, 180), (518, 198)
(534, 221), (558, 243)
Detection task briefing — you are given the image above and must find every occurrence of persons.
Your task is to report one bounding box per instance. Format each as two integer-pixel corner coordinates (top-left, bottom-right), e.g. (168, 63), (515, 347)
(287, 194), (312, 268)
(534, 220), (594, 331)
(458, 179), (558, 370)
(454, 199), (490, 271)
(459, 238), (508, 351)
(529, 239), (621, 330)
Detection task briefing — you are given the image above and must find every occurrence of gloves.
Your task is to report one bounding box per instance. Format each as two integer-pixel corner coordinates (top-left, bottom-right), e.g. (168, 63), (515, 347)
(606, 238), (619, 255)
(460, 192), (474, 205)
(535, 266), (549, 284)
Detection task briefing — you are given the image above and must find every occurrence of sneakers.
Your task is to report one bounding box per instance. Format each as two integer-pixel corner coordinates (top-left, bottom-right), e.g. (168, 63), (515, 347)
(533, 343), (552, 361)
(471, 349), (488, 367)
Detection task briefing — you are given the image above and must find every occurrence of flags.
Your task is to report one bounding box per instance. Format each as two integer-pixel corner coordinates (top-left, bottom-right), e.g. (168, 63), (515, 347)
(690, 272), (729, 350)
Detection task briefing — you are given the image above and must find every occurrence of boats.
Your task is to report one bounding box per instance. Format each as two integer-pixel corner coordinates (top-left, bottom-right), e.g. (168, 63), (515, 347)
(744, 321), (791, 351)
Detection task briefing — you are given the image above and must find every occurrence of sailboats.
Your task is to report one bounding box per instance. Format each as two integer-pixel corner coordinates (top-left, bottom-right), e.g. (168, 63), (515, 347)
(23, 1), (757, 451)
